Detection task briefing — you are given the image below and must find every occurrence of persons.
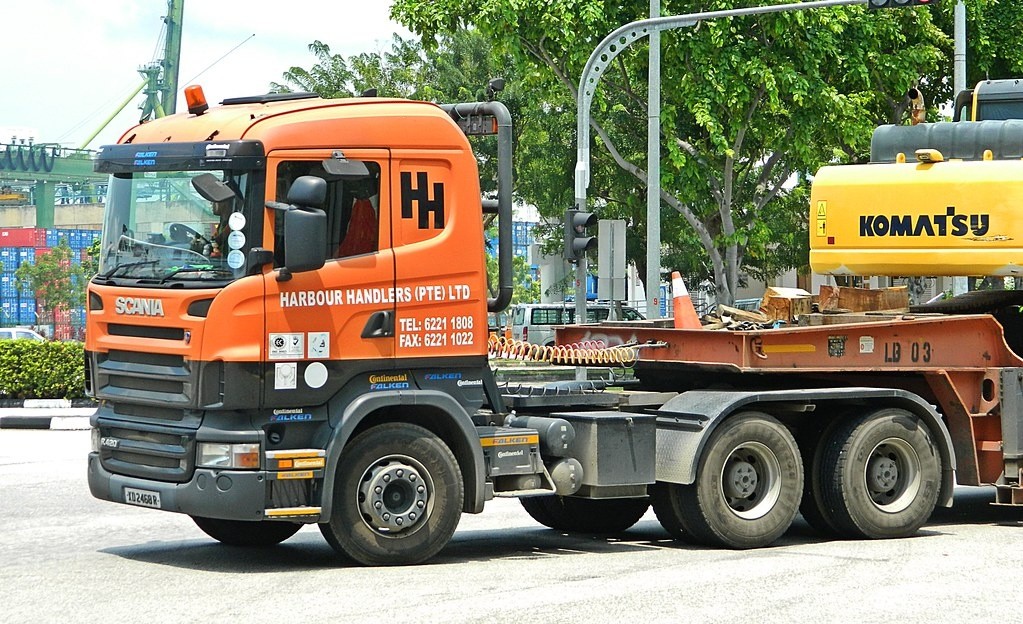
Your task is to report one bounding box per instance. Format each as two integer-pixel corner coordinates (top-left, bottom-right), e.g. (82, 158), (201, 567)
(171, 201), (230, 243)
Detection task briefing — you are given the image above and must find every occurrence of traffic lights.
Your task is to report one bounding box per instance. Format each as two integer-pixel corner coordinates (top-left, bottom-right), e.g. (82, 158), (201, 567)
(564, 210), (598, 260)
(868, 0), (939, 9)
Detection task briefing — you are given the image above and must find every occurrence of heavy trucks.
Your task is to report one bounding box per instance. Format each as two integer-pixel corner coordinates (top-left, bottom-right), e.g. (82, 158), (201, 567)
(87, 85), (1023, 566)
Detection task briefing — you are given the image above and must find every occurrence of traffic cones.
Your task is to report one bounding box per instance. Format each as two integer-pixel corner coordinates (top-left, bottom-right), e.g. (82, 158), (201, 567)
(672, 272), (703, 330)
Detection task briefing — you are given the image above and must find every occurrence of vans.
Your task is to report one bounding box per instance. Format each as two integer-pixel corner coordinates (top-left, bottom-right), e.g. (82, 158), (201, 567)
(512, 304), (646, 359)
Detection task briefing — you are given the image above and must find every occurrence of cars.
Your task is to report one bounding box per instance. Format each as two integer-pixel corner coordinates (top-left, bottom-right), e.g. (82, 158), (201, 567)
(0, 328), (44, 341)
